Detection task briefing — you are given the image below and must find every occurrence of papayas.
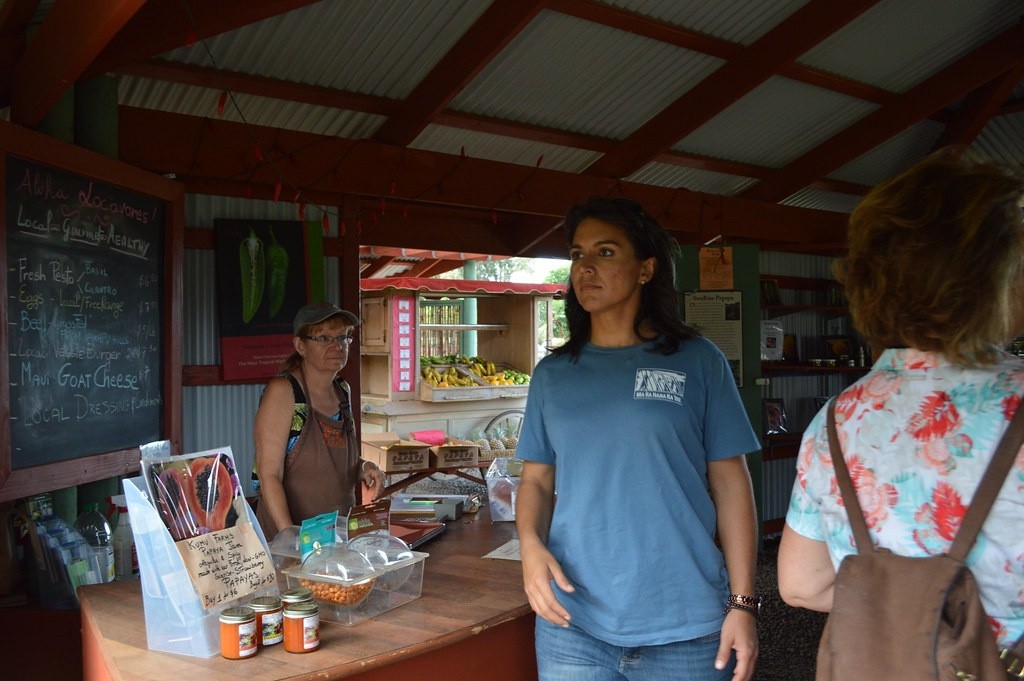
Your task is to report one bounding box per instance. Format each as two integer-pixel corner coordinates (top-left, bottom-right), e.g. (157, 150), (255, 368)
(156, 458), (232, 540)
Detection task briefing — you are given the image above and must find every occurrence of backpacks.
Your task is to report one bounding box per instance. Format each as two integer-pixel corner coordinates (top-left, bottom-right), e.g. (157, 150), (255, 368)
(815, 393), (1024, 681)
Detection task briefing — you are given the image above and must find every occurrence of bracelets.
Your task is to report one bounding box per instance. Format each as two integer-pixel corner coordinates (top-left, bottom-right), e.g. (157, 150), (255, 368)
(723, 601), (759, 615)
(362, 460), (373, 471)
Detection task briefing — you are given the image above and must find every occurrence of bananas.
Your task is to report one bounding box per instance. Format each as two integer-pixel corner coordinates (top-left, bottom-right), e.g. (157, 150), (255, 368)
(422, 362), (496, 387)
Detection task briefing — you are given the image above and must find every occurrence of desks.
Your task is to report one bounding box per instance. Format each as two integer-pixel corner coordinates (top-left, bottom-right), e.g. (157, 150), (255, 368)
(76, 500), (535, 681)
(374, 461), (493, 502)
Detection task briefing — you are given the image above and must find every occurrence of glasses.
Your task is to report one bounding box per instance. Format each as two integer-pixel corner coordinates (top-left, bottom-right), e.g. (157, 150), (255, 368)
(303, 336), (353, 345)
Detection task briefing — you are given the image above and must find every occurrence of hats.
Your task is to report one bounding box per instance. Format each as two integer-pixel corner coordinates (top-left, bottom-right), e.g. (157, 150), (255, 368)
(292, 302), (359, 337)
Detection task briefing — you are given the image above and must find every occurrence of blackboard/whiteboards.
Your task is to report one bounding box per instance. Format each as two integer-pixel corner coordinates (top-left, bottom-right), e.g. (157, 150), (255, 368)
(0, 120), (183, 502)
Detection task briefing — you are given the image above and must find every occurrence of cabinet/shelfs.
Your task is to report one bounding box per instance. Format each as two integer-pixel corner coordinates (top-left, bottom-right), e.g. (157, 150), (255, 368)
(759, 274), (869, 533)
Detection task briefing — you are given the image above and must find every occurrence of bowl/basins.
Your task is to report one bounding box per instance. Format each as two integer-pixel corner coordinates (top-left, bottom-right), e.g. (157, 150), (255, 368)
(823, 359), (836, 367)
(809, 359), (821, 367)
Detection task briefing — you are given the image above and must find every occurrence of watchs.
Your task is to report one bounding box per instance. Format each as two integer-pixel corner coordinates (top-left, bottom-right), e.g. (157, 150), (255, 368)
(729, 595), (763, 614)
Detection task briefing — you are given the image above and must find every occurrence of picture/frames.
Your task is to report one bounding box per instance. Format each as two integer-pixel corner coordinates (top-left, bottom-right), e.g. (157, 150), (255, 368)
(821, 336), (856, 364)
(762, 400), (790, 435)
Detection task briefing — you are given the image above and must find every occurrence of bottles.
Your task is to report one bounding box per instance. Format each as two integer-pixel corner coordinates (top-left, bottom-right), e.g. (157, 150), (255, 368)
(73, 502), (115, 584)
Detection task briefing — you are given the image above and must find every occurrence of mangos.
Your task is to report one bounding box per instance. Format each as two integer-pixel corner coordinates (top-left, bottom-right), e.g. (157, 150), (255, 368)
(482, 373), (515, 386)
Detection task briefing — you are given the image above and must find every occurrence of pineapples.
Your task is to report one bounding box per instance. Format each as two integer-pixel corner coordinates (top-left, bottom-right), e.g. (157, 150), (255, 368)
(457, 420), (520, 453)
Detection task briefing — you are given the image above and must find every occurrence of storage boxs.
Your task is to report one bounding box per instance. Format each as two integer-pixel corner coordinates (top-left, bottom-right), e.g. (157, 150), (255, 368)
(360, 432), (482, 473)
(484, 458), (520, 522)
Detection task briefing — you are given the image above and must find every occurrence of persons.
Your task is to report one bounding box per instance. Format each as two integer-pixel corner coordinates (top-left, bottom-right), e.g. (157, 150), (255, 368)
(775, 141), (1024, 681)
(515, 194), (765, 681)
(256, 298), (388, 543)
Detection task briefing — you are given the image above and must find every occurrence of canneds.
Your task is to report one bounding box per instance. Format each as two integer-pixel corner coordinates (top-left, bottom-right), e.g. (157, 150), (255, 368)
(217, 589), (320, 661)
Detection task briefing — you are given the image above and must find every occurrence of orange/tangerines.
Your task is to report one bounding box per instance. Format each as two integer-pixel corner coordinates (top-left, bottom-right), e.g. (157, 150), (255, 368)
(502, 369), (530, 385)
